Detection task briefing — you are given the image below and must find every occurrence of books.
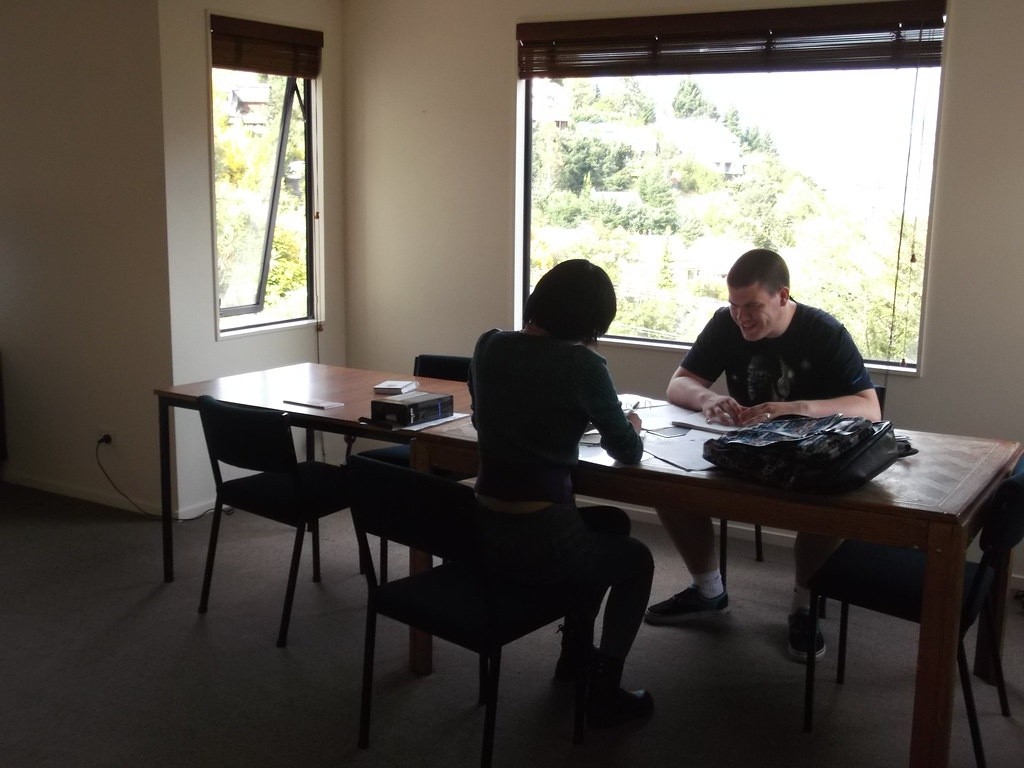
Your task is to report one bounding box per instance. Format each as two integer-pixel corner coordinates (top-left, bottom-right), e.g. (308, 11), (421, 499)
(672, 411), (739, 434)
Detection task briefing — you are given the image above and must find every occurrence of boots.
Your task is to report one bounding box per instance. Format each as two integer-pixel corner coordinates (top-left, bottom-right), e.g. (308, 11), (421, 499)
(589, 656), (653, 731)
(555, 619), (599, 684)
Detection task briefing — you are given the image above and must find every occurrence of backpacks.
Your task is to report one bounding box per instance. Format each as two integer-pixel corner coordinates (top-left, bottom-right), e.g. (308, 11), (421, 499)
(704, 414), (900, 497)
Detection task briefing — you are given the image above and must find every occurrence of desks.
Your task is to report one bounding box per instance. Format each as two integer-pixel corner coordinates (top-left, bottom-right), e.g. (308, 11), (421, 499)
(152, 361), (1024, 768)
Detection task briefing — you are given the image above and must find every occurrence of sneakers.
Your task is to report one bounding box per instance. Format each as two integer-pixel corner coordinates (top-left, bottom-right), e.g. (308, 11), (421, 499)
(788, 610), (827, 665)
(646, 585), (729, 623)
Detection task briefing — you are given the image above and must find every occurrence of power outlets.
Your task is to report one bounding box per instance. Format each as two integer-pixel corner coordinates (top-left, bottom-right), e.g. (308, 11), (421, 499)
(101, 431), (116, 446)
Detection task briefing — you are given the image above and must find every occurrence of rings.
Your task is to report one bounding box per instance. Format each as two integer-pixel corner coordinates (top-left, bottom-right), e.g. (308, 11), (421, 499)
(764, 413), (771, 420)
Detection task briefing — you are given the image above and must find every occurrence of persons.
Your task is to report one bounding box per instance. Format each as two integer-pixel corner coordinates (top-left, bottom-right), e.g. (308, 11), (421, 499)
(644, 249), (883, 662)
(468, 259), (654, 717)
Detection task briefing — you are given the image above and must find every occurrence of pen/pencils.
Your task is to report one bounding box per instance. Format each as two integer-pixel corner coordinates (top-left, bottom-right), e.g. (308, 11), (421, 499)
(626, 402), (640, 418)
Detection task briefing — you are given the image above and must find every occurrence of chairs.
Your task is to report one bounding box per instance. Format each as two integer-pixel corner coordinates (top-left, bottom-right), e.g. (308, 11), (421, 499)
(718, 386), (886, 618)
(342, 454), (589, 768)
(802, 452), (1024, 768)
(199, 395), (388, 648)
(344, 354), (474, 575)
